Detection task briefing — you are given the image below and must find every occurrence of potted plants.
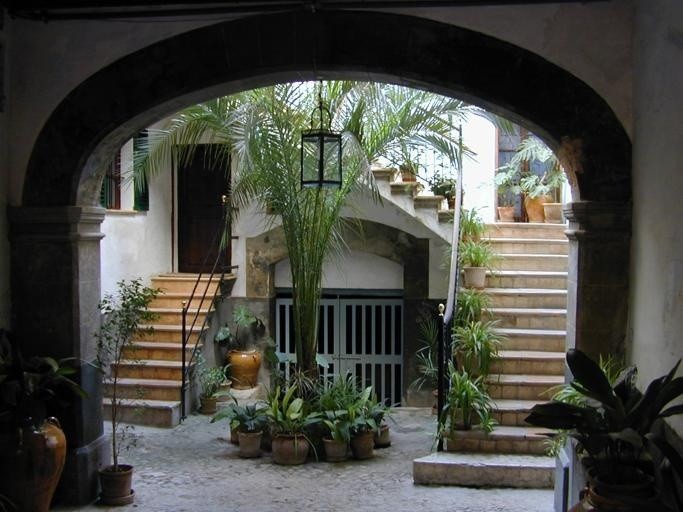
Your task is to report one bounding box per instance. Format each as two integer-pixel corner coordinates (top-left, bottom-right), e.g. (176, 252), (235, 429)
(94, 274), (165, 508)
(453, 205), (488, 245)
(399, 137), (465, 222)
(495, 136), (563, 224)
(199, 303), (392, 464)
(1, 331), (118, 512)
(443, 242), (496, 288)
(407, 307), (445, 401)
(523, 347), (682, 511)
(449, 288), (508, 380)
(442, 372), (496, 429)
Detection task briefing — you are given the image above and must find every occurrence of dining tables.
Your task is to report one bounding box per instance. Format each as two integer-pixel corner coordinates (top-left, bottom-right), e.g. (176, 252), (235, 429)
(199, 303), (392, 464)
(94, 274), (165, 508)
(1, 331), (118, 512)
(523, 347), (682, 511)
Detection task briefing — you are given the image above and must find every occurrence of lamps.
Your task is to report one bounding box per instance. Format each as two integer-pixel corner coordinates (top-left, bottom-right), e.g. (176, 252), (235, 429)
(295, 74), (344, 191)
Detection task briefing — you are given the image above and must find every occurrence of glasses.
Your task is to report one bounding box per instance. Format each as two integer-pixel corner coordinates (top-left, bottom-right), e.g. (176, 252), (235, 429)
(295, 74), (344, 191)
